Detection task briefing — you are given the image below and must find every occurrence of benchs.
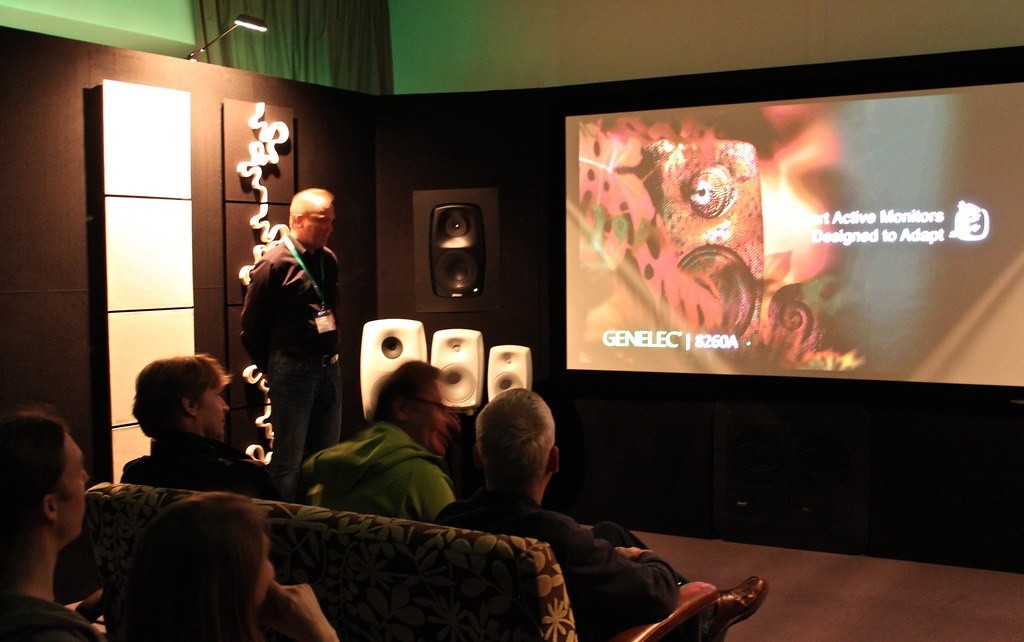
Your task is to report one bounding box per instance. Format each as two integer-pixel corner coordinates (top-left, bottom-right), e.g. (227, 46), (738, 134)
(76, 483), (719, 642)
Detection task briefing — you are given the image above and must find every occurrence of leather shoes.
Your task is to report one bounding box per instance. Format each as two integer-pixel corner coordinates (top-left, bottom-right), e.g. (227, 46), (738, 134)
(703, 576), (769, 642)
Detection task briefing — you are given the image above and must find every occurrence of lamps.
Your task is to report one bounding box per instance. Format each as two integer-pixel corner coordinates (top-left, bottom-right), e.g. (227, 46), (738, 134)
(185, 14), (268, 61)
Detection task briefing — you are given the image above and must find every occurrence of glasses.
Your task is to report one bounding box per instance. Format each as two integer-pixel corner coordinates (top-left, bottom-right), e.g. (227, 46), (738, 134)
(415, 396), (458, 418)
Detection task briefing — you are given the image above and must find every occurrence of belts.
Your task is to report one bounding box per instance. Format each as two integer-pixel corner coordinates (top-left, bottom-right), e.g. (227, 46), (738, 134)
(285, 352), (337, 369)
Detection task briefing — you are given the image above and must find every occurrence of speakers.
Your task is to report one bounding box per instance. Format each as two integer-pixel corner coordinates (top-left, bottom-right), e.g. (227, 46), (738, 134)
(430, 329), (484, 416)
(414, 187), (504, 312)
(360, 318), (427, 419)
(488, 344), (533, 404)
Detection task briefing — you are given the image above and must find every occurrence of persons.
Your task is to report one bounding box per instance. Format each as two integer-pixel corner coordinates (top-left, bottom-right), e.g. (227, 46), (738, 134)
(121, 492), (343, 641)
(238, 188), (346, 510)
(120, 355), (280, 491)
(435, 386), (765, 641)
(0, 408), (112, 642)
(292, 360), (459, 525)
